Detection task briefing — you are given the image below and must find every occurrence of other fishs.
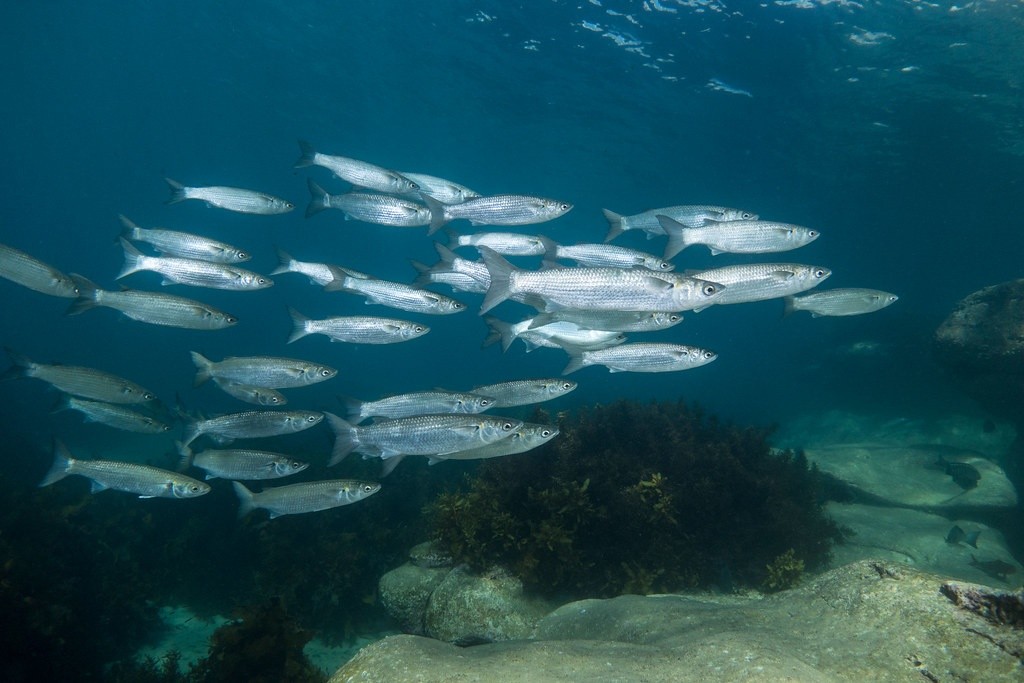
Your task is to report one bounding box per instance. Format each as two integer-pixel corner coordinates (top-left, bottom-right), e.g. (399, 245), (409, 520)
(944, 525), (982, 549)
(0, 137), (900, 527)
(968, 553), (1015, 579)
(933, 453), (982, 485)
